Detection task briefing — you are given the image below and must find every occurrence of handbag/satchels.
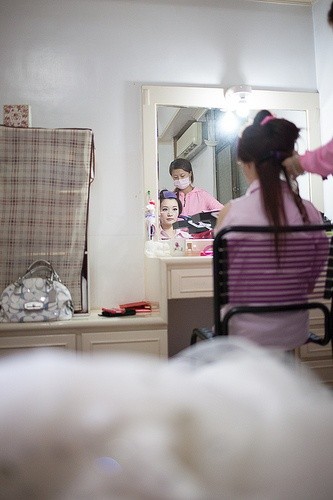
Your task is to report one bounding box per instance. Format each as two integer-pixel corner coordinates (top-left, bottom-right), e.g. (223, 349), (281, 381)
(200, 244), (214, 256)
(0, 259), (74, 323)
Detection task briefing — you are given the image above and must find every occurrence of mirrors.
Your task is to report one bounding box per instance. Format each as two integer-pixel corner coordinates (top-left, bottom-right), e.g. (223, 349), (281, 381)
(142, 86), (324, 254)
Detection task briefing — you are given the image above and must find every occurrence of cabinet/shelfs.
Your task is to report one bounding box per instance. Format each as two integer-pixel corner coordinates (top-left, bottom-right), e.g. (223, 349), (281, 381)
(141, 251), (333, 392)
(0, 311), (168, 372)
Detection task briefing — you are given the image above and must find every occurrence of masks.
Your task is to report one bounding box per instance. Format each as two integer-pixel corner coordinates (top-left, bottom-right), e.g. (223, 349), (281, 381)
(173, 175), (191, 189)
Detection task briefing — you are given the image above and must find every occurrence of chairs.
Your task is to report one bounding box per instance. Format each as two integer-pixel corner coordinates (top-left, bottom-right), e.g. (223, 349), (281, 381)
(188, 224), (333, 366)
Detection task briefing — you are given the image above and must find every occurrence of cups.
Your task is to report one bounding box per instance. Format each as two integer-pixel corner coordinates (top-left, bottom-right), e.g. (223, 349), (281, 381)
(169, 238), (185, 256)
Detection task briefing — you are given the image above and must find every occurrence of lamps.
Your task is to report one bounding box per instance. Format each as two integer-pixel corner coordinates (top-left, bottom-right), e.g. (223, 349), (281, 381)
(227, 87), (251, 116)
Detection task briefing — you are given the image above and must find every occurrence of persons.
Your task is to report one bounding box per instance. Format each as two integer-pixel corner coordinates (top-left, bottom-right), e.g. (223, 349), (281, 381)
(159, 190), (191, 240)
(169, 159), (225, 217)
(214, 110), (329, 351)
(283, 137), (333, 177)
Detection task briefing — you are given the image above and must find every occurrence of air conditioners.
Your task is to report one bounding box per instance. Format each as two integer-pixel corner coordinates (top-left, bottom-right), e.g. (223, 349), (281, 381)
(176, 122), (207, 160)
